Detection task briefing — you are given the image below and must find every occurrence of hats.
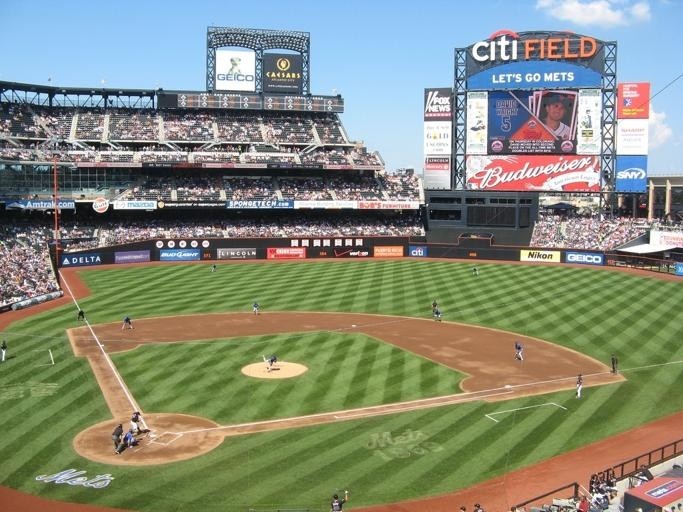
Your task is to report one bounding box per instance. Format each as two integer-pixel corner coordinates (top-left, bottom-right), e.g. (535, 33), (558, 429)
(546, 95), (570, 109)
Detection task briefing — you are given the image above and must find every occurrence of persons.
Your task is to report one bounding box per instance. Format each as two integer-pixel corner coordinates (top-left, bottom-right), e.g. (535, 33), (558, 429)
(0, 98), (386, 170)
(513, 340), (524, 360)
(120, 316), (133, 330)
(265, 355), (276, 371)
(573, 373), (583, 398)
(472, 267), (478, 276)
(472, 503), (484, 512)
(433, 309), (440, 320)
(431, 297), (437, 310)
(110, 424), (121, 454)
(76, 310), (84, 321)
(541, 93), (572, 139)
(0, 339), (6, 361)
(253, 302), (258, 315)
(330, 490), (348, 512)
(130, 411), (142, 433)
(529, 210), (683, 251)
(122, 429), (135, 448)
(610, 352), (617, 375)
(510, 464), (654, 512)
(2, 170), (426, 309)
(459, 505), (466, 511)
